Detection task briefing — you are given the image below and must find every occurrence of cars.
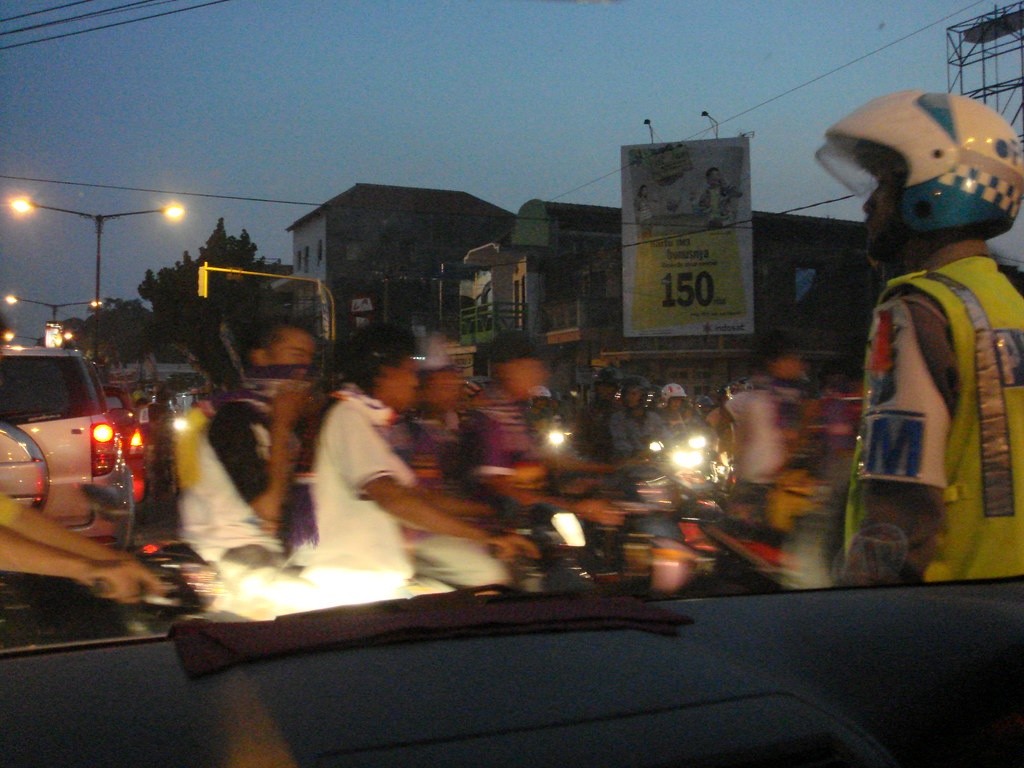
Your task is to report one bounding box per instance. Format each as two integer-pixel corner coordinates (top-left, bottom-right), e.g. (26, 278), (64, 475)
(104, 384), (151, 504)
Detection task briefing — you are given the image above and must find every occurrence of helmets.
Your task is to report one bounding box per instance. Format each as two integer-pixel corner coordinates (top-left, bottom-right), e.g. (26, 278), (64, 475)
(827, 89), (1024, 232)
(530, 385), (552, 406)
(661, 383), (688, 402)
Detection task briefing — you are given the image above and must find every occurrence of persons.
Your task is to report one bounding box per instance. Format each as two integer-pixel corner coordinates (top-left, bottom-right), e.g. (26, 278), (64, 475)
(637, 168), (744, 232)
(816, 90), (1024, 584)
(1, 303), (863, 627)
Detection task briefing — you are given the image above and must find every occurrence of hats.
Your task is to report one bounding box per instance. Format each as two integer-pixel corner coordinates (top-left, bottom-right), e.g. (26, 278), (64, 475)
(621, 374), (651, 390)
(695, 396), (715, 405)
(417, 343), (454, 371)
(344, 323), (414, 367)
(545, 344), (575, 367)
(481, 331), (545, 362)
(593, 367), (623, 387)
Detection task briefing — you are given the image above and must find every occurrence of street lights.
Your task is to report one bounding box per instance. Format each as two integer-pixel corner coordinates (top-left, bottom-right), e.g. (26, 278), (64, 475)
(2, 192), (188, 370)
(4, 293), (103, 350)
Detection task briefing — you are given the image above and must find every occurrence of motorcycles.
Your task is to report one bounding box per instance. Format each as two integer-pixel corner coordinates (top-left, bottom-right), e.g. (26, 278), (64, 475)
(92, 425), (847, 634)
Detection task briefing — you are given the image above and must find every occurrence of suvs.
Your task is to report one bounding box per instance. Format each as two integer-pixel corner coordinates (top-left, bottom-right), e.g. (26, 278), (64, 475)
(0, 350), (138, 556)
